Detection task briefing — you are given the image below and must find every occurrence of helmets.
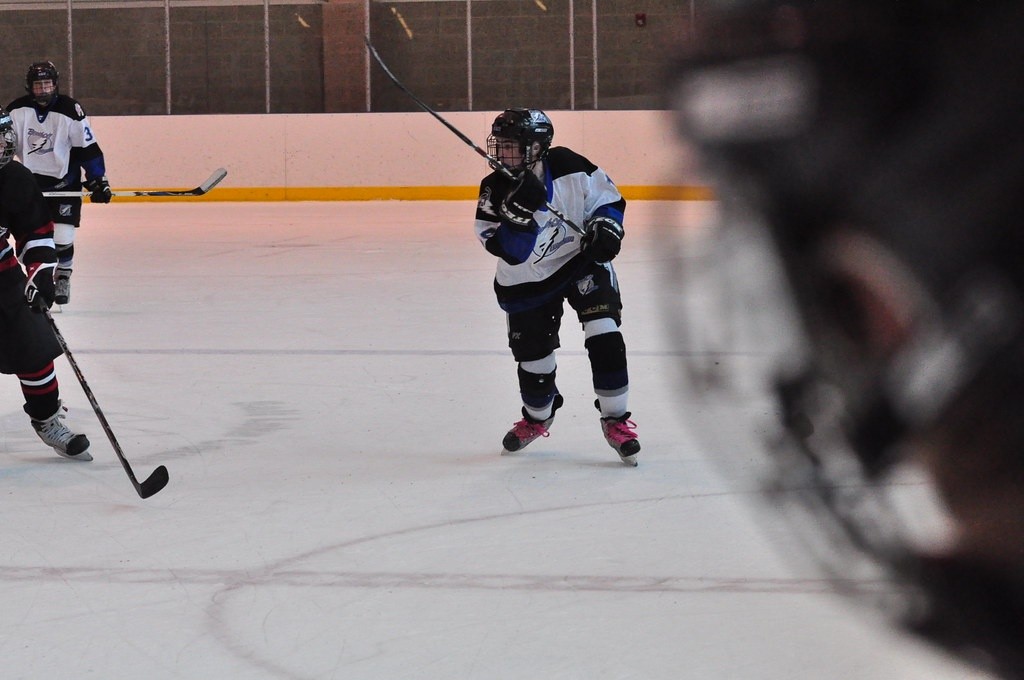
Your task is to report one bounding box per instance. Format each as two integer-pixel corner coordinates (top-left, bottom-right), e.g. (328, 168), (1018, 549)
(492, 107), (554, 161)
(25, 60), (60, 107)
(647, 1), (1024, 679)
(0, 106), (13, 134)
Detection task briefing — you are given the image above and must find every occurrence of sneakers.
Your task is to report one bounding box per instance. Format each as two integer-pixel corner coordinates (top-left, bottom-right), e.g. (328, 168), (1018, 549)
(594, 398), (641, 467)
(23, 399), (93, 461)
(54, 265), (73, 305)
(501, 394), (564, 455)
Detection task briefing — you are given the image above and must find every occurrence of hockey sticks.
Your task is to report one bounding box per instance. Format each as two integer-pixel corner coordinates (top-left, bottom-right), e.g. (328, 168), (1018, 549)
(362, 33), (588, 237)
(38, 294), (171, 499)
(41, 167), (228, 197)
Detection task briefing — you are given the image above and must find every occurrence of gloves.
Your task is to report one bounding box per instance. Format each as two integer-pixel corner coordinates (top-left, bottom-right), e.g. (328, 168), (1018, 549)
(24, 263), (58, 314)
(82, 176), (112, 204)
(498, 169), (548, 230)
(0, 226), (17, 270)
(580, 216), (624, 266)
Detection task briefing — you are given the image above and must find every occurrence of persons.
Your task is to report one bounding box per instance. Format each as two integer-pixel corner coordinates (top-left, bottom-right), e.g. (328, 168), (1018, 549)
(475, 107), (641, 469)
(3, 61), (113, 312)
(0, 108), (93, 461)
(639, 0), (1023, 677)
